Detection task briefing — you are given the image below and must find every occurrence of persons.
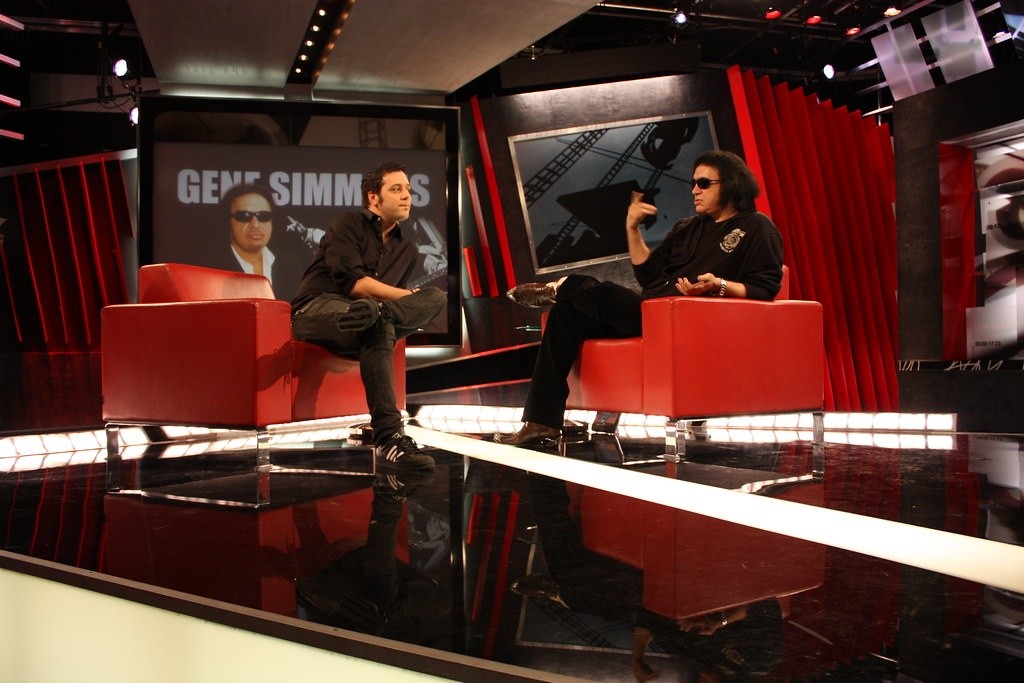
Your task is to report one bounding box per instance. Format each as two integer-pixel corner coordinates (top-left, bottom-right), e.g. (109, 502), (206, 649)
(301, 439), (781, 683)
(208, 184), (301, 303)
(292, 162), (448, 472)
(494, 152), (784, 446)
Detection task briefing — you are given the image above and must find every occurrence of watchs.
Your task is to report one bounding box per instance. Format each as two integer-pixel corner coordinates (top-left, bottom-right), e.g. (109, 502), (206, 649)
(711, 278), (726, 296)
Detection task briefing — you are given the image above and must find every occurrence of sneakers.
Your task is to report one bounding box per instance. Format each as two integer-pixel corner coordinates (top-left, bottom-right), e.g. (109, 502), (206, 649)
(375, 428), (436, 473)
(375, 474), (431, 501)
(334, 297), (389, 333)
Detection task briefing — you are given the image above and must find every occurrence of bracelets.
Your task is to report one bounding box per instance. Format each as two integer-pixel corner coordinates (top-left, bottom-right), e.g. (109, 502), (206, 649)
(411, 289), (415, 293)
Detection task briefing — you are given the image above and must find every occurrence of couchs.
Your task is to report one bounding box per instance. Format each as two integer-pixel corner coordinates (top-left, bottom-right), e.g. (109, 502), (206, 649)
(540, 266), (824, 493)
(100, 265), (404, 511)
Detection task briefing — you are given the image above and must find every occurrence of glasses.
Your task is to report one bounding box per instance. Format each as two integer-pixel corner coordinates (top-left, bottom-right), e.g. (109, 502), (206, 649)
(691, 177), (720, 190)
(228, 210), (274, 222)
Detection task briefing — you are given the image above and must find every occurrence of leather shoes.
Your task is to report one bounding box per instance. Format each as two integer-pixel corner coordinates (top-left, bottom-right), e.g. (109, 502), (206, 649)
(492, 421), (563, 448)
(506, 282), (558, 309)
